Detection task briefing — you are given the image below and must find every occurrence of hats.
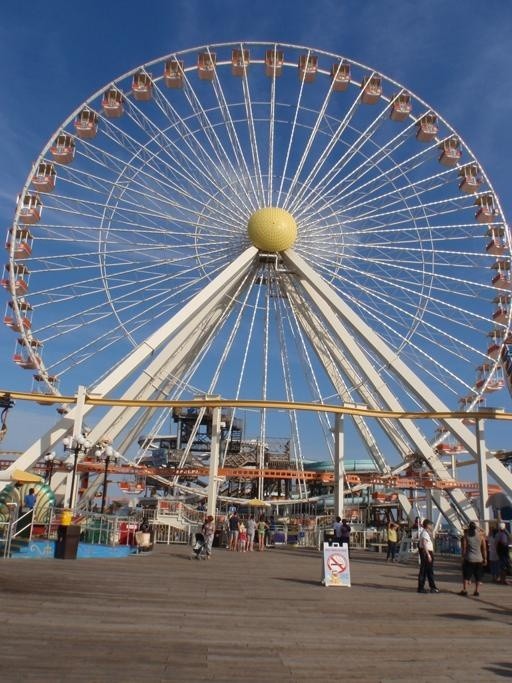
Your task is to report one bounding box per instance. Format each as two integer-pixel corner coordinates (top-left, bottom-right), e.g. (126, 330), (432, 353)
(423, 519), (433, 524)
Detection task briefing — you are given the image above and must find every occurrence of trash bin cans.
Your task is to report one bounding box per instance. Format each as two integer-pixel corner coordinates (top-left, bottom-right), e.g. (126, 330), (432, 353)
(55, 525), (81, 559)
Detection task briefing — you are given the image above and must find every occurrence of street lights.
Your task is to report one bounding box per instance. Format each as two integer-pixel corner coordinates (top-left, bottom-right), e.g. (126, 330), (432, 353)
(43, 433), (121, 523)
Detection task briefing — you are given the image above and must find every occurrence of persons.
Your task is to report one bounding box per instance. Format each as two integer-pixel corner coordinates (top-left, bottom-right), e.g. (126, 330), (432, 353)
(413, 517), (421, 528)
(456, 519), (487, 595)
(340, 518), (350, 559)
(416, 520), (439, 593)
(488, 528), (500, 585)
(222, 510), (276, 554)
(498, 522), (510, 586)
(202, 513), (216, 563)
(197, 502), (207, 511)
(333, 516), (342, 544)
(191, 532), (209, 559)
(23, 488), (38, 508)
(385, 520), (400, 563)
